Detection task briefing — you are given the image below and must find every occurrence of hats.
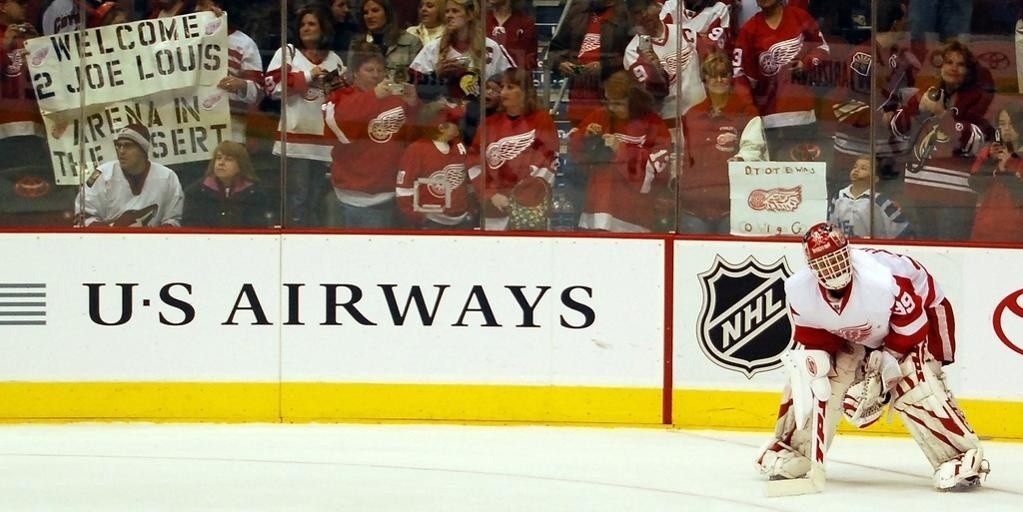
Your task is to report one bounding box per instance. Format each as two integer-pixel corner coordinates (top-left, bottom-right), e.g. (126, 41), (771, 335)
(424, 106), (467, 131)
(116, 122), (150, 151)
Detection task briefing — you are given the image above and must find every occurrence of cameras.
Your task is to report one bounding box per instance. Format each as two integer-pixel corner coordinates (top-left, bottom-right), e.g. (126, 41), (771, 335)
(928, 89), (941, 101)
(572, 64), (584, 74)
(388, 84), (404, 96)
(639, 34), (651, 50)
(17, 24), (26, 34)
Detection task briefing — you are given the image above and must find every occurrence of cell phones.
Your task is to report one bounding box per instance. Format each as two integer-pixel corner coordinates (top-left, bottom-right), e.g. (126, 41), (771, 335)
(992, 128), (1002, 144)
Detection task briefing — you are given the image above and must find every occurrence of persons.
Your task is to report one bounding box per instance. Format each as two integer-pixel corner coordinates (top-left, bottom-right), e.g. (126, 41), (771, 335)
(756, 220), (991, 492)
(548, 2), (1023, 251)
(1, 1), (559, 231)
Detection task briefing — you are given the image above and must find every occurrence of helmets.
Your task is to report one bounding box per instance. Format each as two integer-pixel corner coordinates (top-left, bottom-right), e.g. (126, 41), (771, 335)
(801, 222), (857, 293)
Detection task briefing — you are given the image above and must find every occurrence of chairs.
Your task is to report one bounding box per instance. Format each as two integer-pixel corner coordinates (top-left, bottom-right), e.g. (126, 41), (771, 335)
(516, 3), (592, 234)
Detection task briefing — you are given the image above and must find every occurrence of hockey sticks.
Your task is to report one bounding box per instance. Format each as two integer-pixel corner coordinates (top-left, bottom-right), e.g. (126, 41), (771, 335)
(763, 378), (828, 497)
